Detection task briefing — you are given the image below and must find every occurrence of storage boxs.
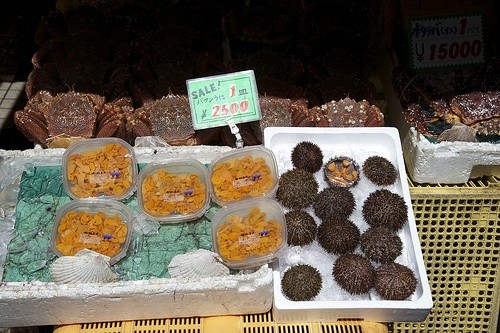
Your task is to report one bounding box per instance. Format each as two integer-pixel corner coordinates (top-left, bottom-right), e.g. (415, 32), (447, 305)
(0, 46), (499, 333)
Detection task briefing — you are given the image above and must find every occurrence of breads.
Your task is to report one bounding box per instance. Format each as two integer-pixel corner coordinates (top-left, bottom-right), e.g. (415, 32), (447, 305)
(13, 0), (385, 149)
(390, 37), (500, 141)
